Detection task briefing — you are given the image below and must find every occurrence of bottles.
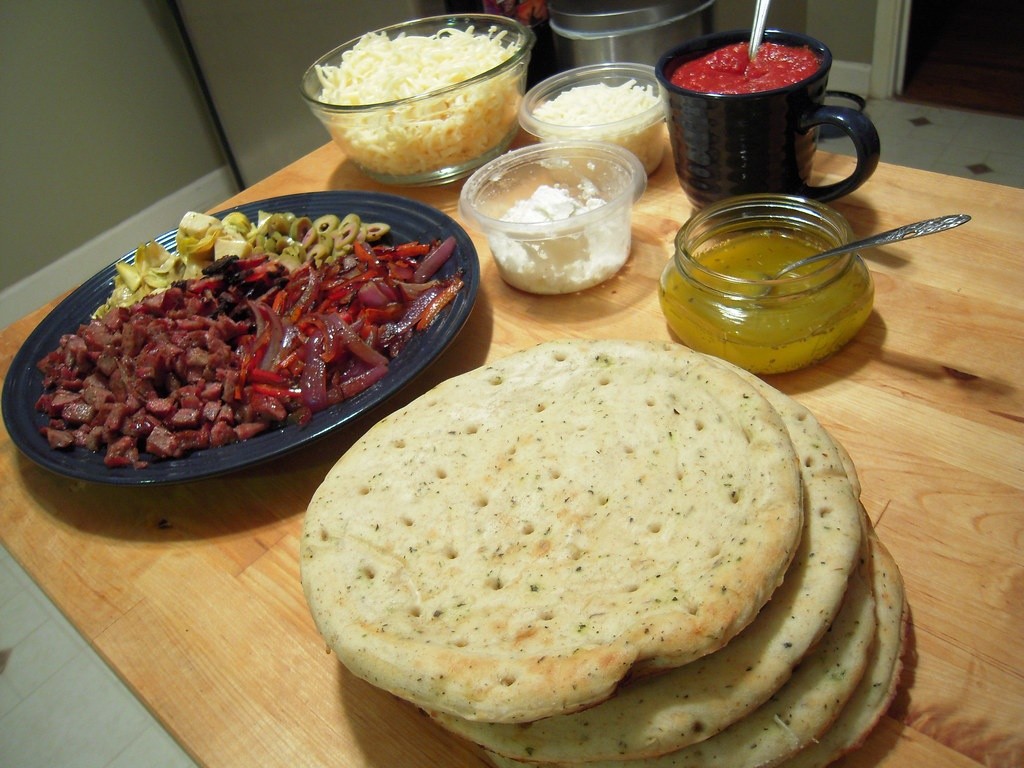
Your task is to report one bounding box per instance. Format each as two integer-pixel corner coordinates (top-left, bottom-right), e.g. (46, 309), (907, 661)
(658, 193), (875, 376)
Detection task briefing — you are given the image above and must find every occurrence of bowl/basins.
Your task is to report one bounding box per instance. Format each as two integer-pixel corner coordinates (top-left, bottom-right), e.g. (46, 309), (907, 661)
(517, 61), (666, 175)
(458, 139), (648, 293)
(298, 14), (536, 185)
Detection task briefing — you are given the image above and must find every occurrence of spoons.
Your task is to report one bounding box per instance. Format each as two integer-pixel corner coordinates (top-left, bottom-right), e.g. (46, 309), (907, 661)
(744, 214), (970, 297)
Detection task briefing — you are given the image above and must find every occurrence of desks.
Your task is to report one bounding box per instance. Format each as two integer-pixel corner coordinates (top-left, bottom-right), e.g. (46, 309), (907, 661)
(0, 96), (1024, 768)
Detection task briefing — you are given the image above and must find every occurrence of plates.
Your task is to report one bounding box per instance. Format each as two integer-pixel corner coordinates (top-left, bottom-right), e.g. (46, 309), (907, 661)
(0, 190), (481, 483)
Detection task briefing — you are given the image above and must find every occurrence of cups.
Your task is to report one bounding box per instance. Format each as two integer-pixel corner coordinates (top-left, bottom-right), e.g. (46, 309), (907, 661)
(651, 27), (883, 203)
